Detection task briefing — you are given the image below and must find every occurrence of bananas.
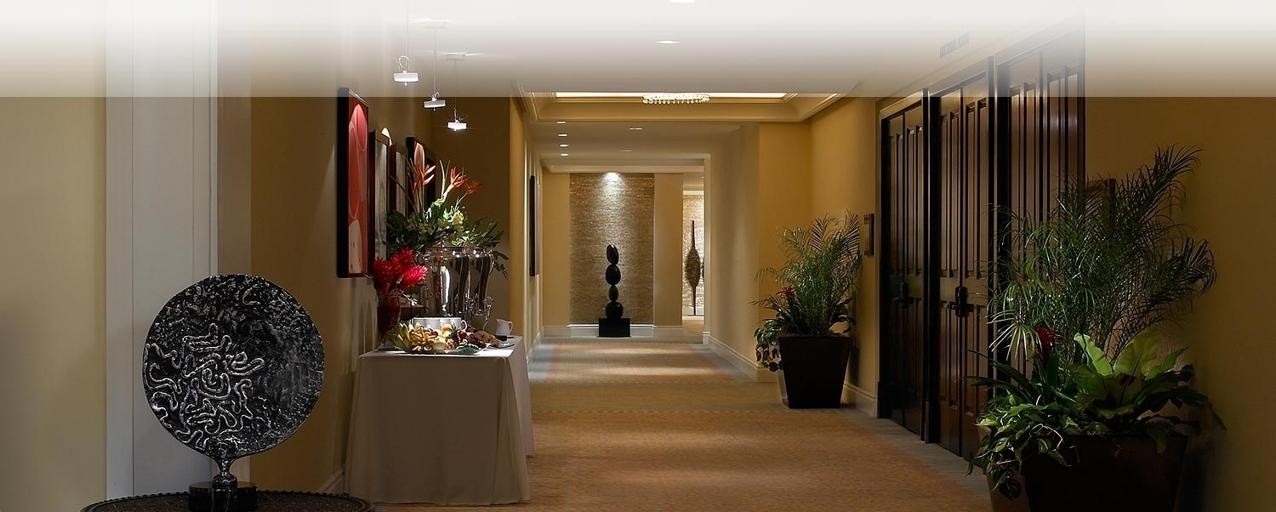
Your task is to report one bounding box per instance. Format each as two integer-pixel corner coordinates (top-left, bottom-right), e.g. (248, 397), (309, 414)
(392, 322), (428, 350)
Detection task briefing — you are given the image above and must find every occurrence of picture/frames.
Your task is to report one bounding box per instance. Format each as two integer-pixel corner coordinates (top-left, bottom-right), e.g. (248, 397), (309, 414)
(406, 136), (436, 219)
(530, 175), (542, 277)
(389, 144), (409, 244)
(368, 130), (391, 276)
(336, 87), (370, 278)
(862, 213), (874, 257)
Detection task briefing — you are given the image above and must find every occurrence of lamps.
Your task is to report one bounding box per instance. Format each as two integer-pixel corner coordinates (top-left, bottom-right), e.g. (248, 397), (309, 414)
(423, 20), (447, 112)
(393, 12), (419, 86)
(444, 53), (468, 132)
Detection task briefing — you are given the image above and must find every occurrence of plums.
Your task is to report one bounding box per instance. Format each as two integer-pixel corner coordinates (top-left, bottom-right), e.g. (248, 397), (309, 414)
(459, 326), (486, 348)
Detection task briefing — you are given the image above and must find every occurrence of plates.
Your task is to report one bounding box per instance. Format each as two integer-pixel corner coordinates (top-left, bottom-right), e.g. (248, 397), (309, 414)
(393, 344), (492, 355)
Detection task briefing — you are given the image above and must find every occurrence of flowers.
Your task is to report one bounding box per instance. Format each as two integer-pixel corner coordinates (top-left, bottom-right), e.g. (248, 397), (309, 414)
(371, 247), (429, 299)
(391, 141), (484, 246)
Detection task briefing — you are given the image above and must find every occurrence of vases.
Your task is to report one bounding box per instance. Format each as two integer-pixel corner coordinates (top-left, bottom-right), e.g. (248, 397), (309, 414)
(416, 229), (469, 317)
(376, 288), (401, 351)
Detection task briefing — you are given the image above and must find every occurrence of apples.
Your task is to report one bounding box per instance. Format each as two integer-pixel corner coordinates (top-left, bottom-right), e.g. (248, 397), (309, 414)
(433, 323), (453, 341)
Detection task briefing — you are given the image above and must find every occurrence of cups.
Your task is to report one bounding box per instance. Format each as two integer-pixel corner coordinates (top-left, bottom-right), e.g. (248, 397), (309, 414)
(496, 318), (514, 337)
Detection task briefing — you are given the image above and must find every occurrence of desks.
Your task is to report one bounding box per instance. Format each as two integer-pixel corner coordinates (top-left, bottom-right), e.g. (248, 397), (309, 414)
(343, 333), (537, 506)
(81, 491), (371, 512)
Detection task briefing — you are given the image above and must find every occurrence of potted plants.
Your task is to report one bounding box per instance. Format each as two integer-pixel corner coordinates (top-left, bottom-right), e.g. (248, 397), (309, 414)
(745, 208), (863, 410)
(965, 143), (1229, 512)
(468, 216), (510, 317)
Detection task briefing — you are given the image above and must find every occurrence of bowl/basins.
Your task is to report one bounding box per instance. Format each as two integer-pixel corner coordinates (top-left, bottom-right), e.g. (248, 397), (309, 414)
(411, 318), (467, 342)
